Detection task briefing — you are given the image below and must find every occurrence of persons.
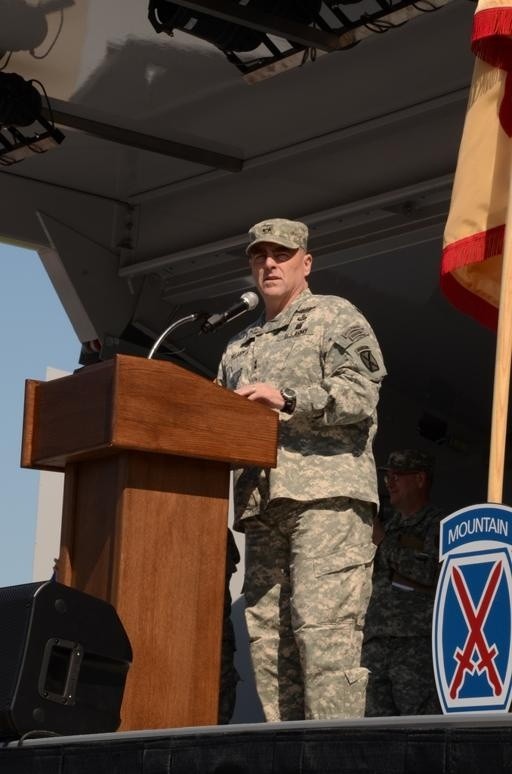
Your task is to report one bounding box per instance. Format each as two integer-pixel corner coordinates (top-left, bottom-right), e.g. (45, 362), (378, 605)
(211, 219), (386, 722)
(365, 448), (450, 717)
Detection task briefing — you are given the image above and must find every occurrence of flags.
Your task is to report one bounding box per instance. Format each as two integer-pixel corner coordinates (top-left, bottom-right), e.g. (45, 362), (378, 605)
(440, 0), (512, 326)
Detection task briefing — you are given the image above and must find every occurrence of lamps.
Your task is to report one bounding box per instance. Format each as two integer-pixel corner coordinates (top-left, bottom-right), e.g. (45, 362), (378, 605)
(145, 1), (455, 87)
(0, 71), (66, 167)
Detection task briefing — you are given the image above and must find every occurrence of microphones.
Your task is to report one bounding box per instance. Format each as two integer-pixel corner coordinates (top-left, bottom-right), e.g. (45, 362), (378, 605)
(197, 292), (258, 336)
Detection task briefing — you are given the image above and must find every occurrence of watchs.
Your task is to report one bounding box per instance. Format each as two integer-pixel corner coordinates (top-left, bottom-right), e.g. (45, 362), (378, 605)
(280, 387), (297, 413)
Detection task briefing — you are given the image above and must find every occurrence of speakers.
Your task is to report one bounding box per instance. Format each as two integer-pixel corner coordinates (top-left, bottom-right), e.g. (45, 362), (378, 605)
(0, 580), (132, 742)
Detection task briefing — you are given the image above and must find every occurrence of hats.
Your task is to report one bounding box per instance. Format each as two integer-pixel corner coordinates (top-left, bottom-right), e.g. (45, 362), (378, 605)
(245, 218), (308, 256)
